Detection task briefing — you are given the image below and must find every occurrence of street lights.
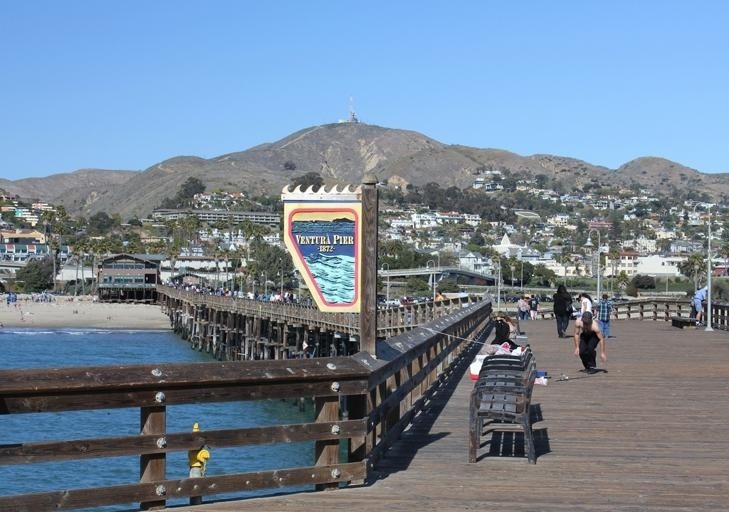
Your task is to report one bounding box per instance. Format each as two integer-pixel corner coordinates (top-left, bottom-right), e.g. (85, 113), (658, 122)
(692, 202), (716, 333)
(511, 260), (524, 291)
(381, 263), (389, 308)
(585, 228), (600, 304)
(426, 260), (436, 302)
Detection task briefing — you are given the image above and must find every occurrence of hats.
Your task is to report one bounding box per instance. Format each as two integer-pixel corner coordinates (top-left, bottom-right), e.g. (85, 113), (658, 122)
(581, 311), (592, 323)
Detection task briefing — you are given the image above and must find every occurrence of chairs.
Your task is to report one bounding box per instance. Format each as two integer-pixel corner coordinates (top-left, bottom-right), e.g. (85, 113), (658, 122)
(467, 344), (538, 464)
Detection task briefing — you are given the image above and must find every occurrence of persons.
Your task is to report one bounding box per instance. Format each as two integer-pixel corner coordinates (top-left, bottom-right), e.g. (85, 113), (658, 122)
(517, 294), (540, 320)
(574, 312), (606, 374)
(491, 311), (517, 353)
(578, 293), (592, 316)
(435, 287), (444, 300)
(553, 285), (573, 338)
(162, 278), (312, 305)
(596, 293), (612, 339)
(690, 285), (708, 326)
